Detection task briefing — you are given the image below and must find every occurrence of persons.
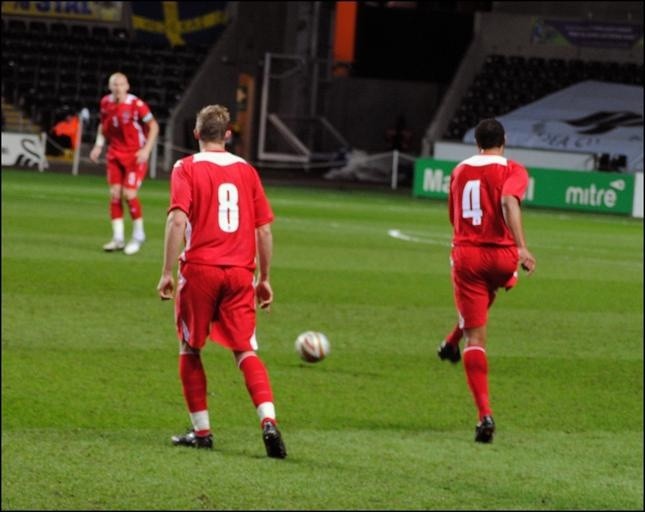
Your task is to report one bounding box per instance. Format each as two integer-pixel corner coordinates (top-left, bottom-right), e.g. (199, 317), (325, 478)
(436, 118), (535, 442)
(156, 103), (288, 460)
(91, 70), (160, 255)
(46, 104), (80, 156)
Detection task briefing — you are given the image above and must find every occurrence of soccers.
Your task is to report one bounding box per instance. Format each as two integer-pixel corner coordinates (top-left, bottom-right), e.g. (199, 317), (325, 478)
(294, 329), (329, 363)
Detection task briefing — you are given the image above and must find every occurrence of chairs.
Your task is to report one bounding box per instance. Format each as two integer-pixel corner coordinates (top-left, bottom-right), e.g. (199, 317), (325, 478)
(442, 53), (645, 142)
(0, 18), (221, 154)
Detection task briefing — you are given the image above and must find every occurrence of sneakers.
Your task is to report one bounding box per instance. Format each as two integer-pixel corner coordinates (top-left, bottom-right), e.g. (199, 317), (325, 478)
(263, 423), (287, 458)
(473, 416), (495, 442)
(172, 433), (213, 448)
(103, 238), (125, 252)
(122, 236), (145, 255)
(439, 341), (460, 363)
